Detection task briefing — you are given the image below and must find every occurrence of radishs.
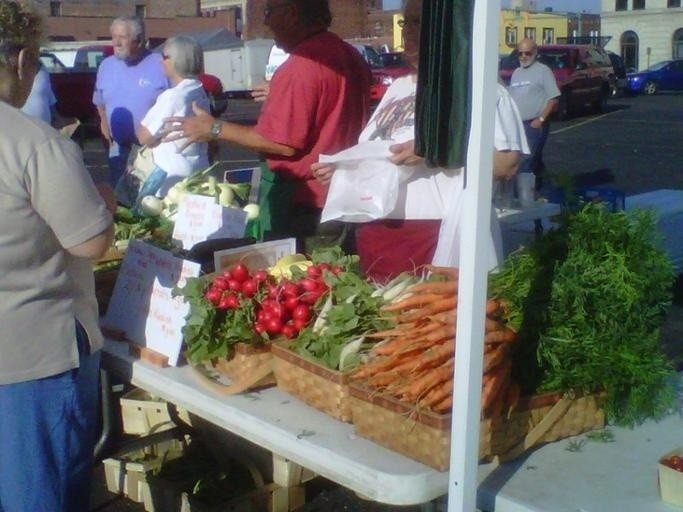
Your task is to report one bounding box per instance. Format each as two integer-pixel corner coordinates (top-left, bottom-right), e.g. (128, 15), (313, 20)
(311, 264), (431, 371)
(140, 162), (262, 243)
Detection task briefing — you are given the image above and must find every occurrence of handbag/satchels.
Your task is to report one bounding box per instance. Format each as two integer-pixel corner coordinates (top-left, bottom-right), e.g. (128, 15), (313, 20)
(199, 73), (227, 116)
(112, 149), (167, 214)
(305, 223), (353, 257)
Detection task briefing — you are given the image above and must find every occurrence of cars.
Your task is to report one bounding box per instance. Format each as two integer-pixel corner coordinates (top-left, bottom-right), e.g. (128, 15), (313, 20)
(37, 49), (69, 71)
(364, 48), (418, 104)
(626, 59), (680, 96)
(605, 49), (627, 96)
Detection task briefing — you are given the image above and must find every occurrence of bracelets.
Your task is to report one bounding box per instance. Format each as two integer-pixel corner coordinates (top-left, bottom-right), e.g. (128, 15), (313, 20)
(210, 117), (223, 140)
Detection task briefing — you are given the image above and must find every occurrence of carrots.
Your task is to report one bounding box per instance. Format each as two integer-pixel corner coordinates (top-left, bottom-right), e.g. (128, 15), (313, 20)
(350, 265), (520, 414)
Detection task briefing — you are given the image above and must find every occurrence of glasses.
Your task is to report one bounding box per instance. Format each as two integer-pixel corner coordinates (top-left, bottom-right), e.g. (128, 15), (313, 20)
(264, 3), (288, 15)
(161, 51), (170, 60)
(26, 52), (43, 73)
(398, 18), (420, 26)
(518, 51), (535, 56)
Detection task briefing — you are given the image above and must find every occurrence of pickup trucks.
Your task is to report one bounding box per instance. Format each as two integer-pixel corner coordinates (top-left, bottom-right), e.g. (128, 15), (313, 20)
(39, 44), (228, 133)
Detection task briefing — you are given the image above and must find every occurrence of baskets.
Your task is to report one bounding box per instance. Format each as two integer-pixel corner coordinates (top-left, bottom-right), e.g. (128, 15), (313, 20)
(350, 381), (606, 471)
(186, 338), (286, 395)
(95, 269), (118, 315)
(272, 339), (361, 422)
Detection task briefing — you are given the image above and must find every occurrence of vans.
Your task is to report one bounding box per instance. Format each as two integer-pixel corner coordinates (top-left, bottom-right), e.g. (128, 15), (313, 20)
(262, 42), (390, 111)
(499, 45), (616, 114)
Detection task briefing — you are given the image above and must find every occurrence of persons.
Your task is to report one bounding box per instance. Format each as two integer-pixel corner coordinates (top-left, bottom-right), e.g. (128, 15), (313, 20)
(92, 14), (176, 186)
(307, 0), (532, 284)
(19, 62), (65, 126)
(157, 0), (373, 254)
(134, 33), (212, 196)
(1, 0), (121, 512)
(507, 36), (563, 198)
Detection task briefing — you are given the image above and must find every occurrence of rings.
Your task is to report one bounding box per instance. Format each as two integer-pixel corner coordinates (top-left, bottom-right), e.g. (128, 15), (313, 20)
(178, 130), (184, 139)
